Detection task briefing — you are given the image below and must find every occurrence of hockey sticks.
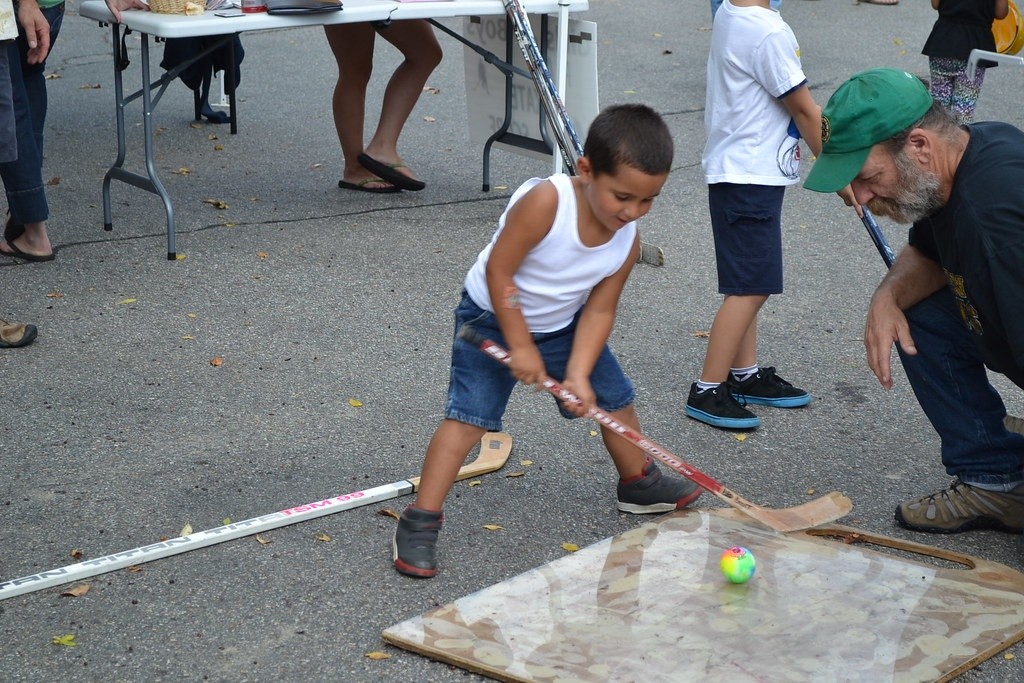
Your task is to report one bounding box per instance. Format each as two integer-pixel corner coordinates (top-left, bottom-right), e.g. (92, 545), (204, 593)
(458, 325), (851, 531)
(858, 203), (899, 268)
(503, 0), (664, 267)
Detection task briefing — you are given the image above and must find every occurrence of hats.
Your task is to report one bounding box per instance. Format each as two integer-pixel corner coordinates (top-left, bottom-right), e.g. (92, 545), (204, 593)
(801, 68), (935, 194)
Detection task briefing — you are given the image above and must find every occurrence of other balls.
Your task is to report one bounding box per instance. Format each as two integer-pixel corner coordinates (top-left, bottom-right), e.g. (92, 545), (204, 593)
(721, 544), (757, 583)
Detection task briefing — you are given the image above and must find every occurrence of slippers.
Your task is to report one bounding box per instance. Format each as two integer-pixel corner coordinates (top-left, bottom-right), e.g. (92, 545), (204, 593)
(357, 153), (425, 191)
(3, 232), (56, 263)
(337, 176), (401, 192)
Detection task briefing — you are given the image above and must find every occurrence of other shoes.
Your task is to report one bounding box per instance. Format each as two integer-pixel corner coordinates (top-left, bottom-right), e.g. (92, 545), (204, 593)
(3, 321), (35, 345)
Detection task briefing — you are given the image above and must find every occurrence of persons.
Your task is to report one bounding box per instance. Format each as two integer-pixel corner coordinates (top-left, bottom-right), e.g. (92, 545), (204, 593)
(323, 18), (442, 192)
(921, 0), (1008, 124)
(392, 103), (702, 579)
(0, 0), (149, 347)
(688, 0), (865, 429)
(803, 67), (1024, 532)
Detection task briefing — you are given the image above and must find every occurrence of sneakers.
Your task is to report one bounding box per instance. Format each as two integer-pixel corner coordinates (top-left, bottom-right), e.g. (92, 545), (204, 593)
(686, 381), (760, 427)
(730, 368), (812, 408)
(393, 506), (444, 578)
(896, 479), (1022, 535)
(614, 460), (702, 515)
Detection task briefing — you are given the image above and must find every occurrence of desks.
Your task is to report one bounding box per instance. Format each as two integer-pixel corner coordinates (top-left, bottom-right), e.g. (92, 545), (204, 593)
(77, 0), (590, 262)
